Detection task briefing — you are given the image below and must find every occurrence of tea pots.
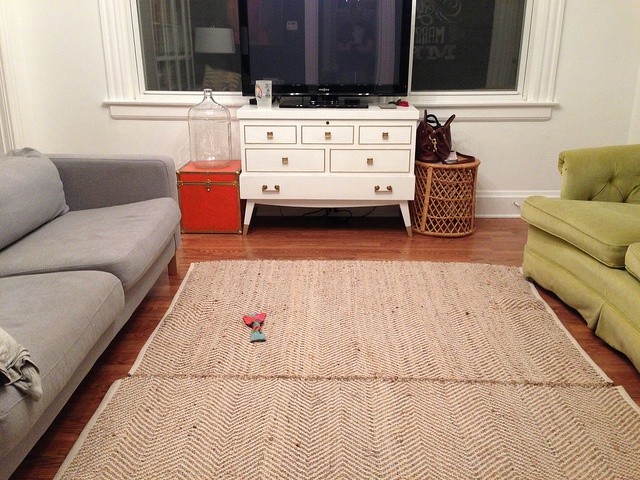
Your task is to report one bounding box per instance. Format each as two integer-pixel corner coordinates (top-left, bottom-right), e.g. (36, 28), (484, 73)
(134, 1), (239, 95)
(410, 1), (527, 96)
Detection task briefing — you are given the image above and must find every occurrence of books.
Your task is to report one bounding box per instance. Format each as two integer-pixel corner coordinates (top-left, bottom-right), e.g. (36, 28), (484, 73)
(175, 158), (240, 238)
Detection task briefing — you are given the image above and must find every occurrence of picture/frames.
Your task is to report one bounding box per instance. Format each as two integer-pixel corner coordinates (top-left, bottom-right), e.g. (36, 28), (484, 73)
(239, 99), (420, 237)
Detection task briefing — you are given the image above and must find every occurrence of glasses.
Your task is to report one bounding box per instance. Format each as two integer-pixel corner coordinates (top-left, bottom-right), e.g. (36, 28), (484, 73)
(192, 22), (237, 93)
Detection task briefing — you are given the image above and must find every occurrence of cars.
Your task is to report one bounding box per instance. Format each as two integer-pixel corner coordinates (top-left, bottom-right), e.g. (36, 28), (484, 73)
(255, 79), (272, 111)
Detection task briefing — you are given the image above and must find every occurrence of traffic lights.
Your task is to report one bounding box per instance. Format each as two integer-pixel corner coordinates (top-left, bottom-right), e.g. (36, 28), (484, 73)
(202, 63), (241, 96)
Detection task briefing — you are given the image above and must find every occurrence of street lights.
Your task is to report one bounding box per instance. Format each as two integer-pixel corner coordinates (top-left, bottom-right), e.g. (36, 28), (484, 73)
(416, 109), (456, 162)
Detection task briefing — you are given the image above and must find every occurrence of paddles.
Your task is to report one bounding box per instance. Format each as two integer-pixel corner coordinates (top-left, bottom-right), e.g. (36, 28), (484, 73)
(52, 259), (638, 480)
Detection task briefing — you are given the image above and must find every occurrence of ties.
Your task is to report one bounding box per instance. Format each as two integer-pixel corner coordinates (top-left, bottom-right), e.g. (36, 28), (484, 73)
(237, 0), (412, 109)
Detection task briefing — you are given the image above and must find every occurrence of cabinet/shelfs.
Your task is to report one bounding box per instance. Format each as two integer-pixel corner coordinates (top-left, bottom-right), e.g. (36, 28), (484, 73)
(187, 90), (231, 168)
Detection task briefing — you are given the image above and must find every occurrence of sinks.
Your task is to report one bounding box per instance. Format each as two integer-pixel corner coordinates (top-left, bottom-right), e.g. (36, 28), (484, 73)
(518, 144), (639, 413)
(0, 146), (183, 479)
